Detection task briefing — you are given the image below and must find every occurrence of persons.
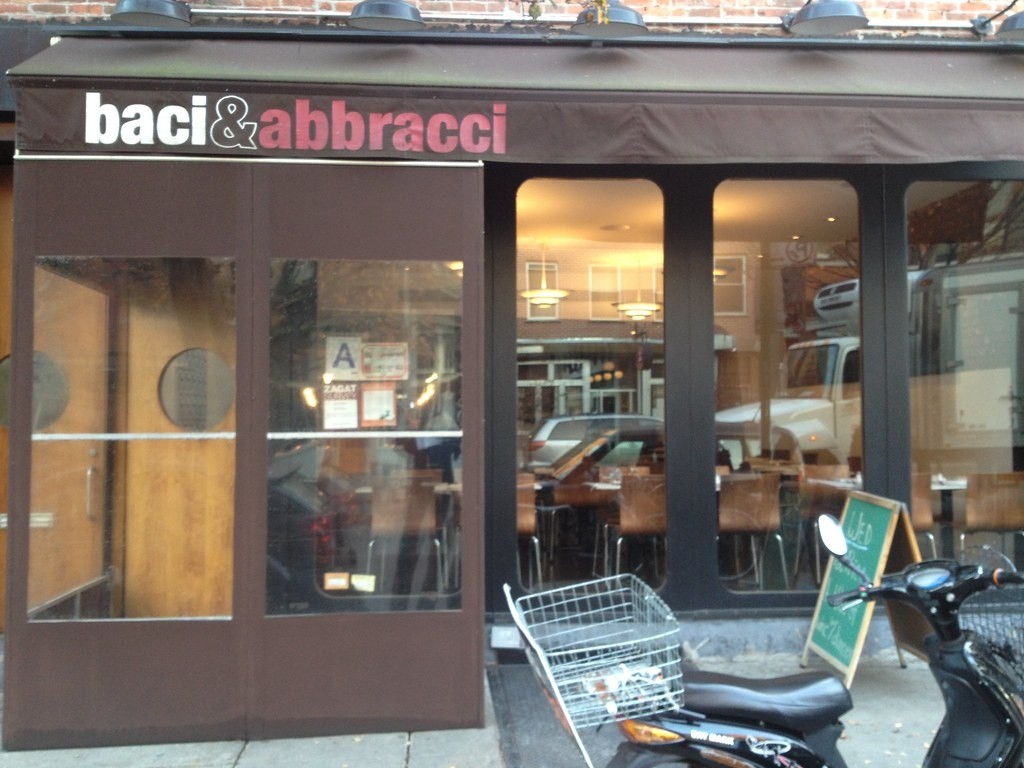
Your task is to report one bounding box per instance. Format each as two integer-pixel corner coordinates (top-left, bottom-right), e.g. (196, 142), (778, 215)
(386, 344), (868, 609)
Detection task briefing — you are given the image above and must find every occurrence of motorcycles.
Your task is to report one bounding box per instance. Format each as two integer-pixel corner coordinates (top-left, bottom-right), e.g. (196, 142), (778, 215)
(574, 510), (1024, 767)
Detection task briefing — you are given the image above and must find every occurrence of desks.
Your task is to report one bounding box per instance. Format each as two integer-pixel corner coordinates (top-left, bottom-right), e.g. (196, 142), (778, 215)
(354, 483), (544, 494)
(806, 476), (968, 491)
(582, 475), (720, 494)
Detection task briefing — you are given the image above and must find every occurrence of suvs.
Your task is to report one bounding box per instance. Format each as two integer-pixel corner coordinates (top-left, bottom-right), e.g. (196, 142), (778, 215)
(433, 420), (809, 537)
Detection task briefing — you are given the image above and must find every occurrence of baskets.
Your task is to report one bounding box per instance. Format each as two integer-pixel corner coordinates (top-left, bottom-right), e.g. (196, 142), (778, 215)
(957, 545), (1024, 695)
(516, 573), (685, 729)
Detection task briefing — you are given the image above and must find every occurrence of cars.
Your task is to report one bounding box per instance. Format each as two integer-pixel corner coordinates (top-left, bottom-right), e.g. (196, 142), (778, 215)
(284, 430), (460, 597)
(524, 414), (666, 473)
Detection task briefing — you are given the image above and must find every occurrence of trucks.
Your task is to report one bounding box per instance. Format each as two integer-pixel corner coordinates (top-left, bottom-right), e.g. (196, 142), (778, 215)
(714, 252), (1022, 475)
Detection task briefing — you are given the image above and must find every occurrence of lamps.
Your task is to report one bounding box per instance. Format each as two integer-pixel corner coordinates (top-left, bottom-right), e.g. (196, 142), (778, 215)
(109, 0), (193, 31)
(519, 242), (569, 311)
(610, 254), (662, 322)
(781, 0), (870, 36)
(972, 0), (1023, 40)
(345, 0), (426, 32)
(570, 0), (649, 37)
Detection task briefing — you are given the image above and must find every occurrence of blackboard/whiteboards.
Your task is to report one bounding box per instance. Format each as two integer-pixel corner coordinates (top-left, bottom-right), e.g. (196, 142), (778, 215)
(807, 488), (900, 677)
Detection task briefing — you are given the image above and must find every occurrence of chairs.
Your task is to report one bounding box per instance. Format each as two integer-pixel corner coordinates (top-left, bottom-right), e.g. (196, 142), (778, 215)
(311, 461), (1023, 601)
